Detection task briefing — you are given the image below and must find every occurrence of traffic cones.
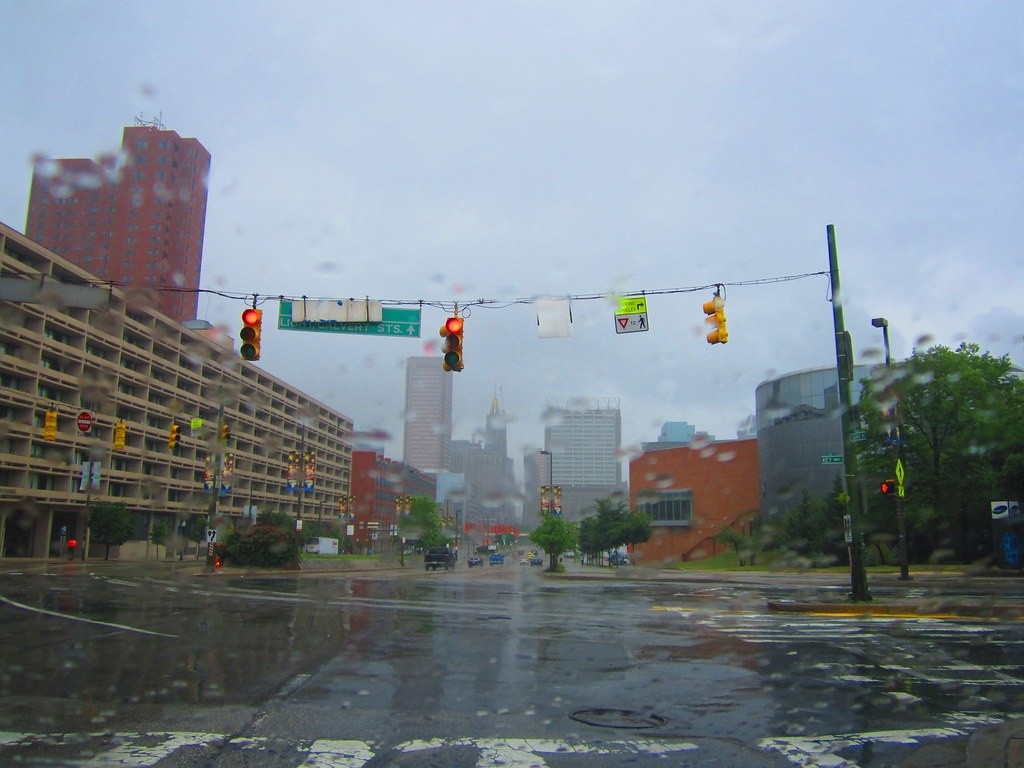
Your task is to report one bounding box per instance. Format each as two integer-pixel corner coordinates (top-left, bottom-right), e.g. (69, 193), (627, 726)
(214, 555), (222, 574)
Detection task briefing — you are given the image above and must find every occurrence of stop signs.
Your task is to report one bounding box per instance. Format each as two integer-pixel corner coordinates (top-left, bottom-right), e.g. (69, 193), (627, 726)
(76, 411), (92, 434)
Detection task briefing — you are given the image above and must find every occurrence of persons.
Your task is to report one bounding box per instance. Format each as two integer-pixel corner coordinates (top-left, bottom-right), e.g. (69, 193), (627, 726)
(581, 551), (584, 565)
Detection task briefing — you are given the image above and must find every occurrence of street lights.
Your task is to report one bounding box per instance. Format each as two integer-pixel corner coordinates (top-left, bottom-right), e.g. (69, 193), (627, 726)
(446, 499), (452, 525)
(540, 450), (553, 572)
(318, 500), (328, 538)
(455, 509), (461, 560)
(872, 317), (913, 580)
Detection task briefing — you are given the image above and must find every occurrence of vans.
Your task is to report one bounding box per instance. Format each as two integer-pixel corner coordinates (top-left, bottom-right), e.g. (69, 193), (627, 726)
(305, 538), (321, 554)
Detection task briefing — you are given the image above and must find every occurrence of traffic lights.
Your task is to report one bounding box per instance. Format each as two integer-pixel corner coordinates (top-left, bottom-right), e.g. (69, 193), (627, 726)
(67, 539), (77, 548)
(239, 308), (263, 362)
(222, 425), (231, 446)
(168, 426), (182, 452)
(704, 296), (727, 343)
(880, 478), (898, 495)
(440, 315), (464, 373)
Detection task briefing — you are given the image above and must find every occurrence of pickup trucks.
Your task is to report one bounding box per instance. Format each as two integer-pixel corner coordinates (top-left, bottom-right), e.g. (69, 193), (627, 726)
(425, 547), (456, 571)
(564, 550), (575, 558)
(489, 554), (504, 565)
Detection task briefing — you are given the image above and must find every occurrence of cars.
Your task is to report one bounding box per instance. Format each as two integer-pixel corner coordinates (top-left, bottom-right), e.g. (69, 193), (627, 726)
(601, 552), (627, 565)
(520, 557), (529, 565)
(531, 557), (543, 566)
(469, 555), (483, 567)
(513, 546), (538, 558)
